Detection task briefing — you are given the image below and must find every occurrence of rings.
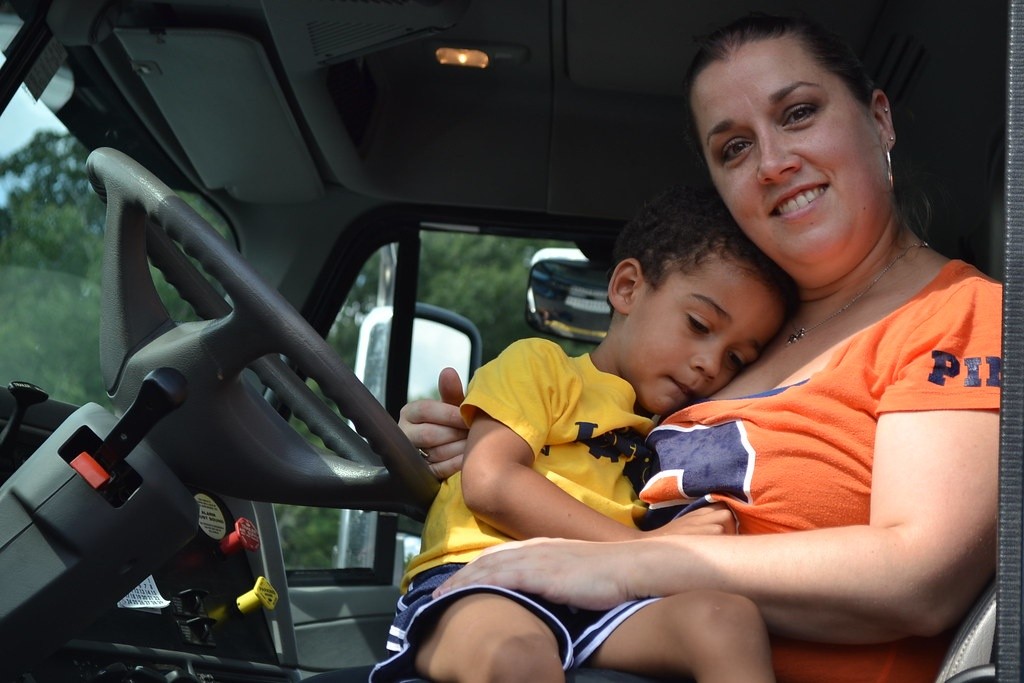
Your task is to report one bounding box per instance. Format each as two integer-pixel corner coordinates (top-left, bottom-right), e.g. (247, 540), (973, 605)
(419, 448), (429, 459)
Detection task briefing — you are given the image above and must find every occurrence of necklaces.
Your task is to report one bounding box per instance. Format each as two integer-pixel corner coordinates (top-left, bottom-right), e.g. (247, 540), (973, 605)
(786, 242), (929, 345)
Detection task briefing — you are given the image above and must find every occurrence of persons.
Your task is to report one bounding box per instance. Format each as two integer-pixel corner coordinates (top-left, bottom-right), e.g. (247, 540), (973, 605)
(396, 13), (1003, 683)
(369, 181), (801, 683)
